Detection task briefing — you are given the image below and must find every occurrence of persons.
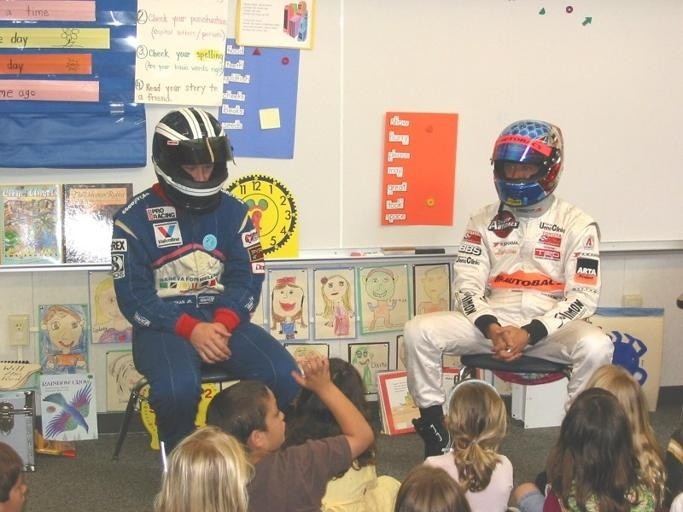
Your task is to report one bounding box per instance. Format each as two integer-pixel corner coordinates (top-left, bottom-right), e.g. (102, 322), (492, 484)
(425, 381), (513, 512)
(402, 120), (614, 457)
(515, 366), (683, 512)
(205, 354), (402, 512)
(110, 107), (303, 453)
(161, 426), (256, 512)
(395, 465), (471, 512)
(0, 443), (28, 512)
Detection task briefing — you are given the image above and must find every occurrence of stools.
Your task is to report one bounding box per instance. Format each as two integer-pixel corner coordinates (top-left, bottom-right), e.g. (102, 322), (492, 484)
(110, 349), (235, 463)
(453, 355), (574, 391)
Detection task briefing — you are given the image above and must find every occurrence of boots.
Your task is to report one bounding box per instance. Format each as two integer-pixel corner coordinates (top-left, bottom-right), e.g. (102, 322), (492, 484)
(411, 417), (453, 460)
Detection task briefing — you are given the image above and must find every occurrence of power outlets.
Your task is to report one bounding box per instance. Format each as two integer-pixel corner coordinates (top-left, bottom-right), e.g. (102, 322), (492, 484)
(8, 315), (29, 347)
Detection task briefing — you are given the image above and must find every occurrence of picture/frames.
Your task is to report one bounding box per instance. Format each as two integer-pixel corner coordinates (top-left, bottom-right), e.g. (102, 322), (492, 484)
(413, 262), (451, 316)
(61, 181), (135, 266)
(396, 334), (406, 371)
(347, 341), (391, 395)
(283, 342), (331, 378)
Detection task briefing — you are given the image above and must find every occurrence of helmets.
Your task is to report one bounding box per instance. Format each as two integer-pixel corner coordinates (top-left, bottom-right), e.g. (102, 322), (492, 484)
(152, 107), (236, 214)
(491, 121), (563, 209)
